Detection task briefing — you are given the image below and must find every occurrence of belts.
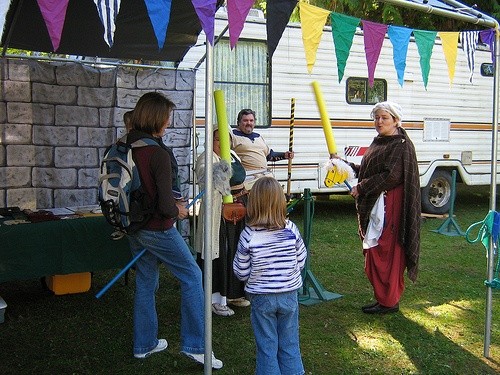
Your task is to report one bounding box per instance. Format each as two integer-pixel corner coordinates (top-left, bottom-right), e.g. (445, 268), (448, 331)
(231, 189), (250, 199)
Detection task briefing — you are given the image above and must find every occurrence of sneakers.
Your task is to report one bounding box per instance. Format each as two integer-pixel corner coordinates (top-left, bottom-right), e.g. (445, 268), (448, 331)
(181, 351), (222, 368)
(134, 339), (168, 358)
(211, 302), (234, 316)
(227, 296), (250, 306)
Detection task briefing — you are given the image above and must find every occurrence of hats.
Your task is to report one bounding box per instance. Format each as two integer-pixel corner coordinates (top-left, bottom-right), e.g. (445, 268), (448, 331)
(370, 102), (402, 127)
(213, 123), (234, 141)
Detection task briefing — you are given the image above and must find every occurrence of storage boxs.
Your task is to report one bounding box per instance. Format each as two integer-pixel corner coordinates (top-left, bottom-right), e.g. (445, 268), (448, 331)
(46, 272), (91, 295)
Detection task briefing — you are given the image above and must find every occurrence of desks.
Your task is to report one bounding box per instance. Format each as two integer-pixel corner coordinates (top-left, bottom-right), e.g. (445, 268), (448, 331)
(0, 214), (135, 285)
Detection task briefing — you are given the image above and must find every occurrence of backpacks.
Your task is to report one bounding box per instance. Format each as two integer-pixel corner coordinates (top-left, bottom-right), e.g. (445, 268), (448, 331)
(97, 135), (160, 241)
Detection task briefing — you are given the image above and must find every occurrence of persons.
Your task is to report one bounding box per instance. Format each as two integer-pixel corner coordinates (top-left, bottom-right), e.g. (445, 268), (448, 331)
(233, 177), (308, 375)
(196, 122), (251, 315)
(231, 109), (294, 194)
(348, 100), (420, 315)
(104, 91), (224, 368)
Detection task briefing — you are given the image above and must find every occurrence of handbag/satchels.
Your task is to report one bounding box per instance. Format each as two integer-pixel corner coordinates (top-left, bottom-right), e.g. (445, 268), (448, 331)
(0, 207), (20, 217)
(26, 210), (61, 223)
(221, 202), (247, 225)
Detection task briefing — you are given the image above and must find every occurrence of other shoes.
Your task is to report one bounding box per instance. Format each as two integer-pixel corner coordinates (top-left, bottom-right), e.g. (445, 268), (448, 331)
(362, 302), (399, 314)
